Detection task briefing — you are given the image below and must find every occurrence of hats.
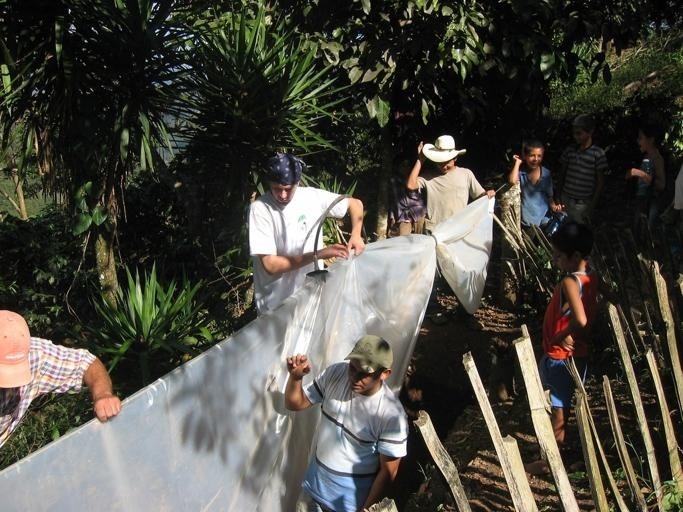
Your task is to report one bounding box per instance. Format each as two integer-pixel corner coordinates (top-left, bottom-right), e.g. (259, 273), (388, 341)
(551, 220), (595, 248)
(344, 334), (394, 374)
(422, 134), (467, 164)
(261, 152), (303, 185)
(572, 114), (597, 133)
(0, 309), (33, 389)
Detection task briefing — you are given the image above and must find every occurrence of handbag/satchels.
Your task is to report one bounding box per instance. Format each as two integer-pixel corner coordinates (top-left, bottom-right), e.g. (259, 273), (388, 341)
(640, 186), (656, 222)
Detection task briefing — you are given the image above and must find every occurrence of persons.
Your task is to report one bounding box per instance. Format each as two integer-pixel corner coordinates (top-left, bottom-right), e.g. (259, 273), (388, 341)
(249, 152), (366, 314)
(525, 223), (599, 476)
(0, 307), (121, 459)
(389, 107), (683, 309)
(284, 335), (408, 511)
